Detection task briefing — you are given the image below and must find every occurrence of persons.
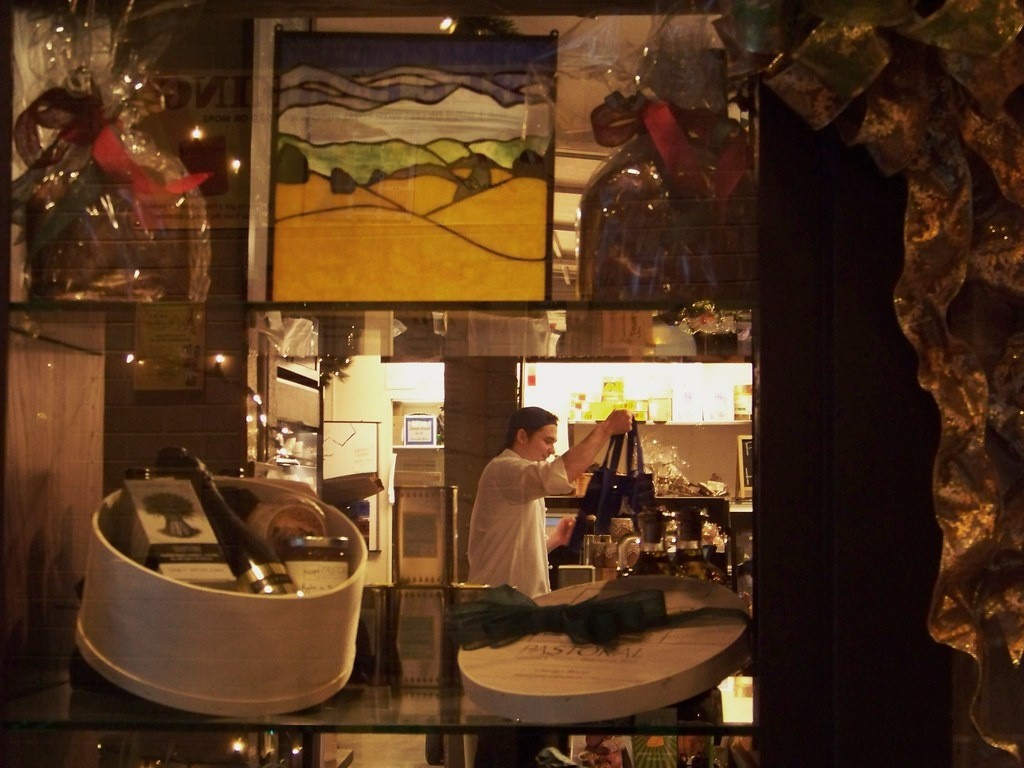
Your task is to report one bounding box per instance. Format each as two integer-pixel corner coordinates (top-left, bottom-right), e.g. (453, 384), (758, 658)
(459, 408), (632, 763)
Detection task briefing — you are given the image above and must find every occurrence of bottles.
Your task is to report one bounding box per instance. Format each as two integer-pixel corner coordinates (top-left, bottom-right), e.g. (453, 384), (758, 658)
(171, 457), (297, 596)
